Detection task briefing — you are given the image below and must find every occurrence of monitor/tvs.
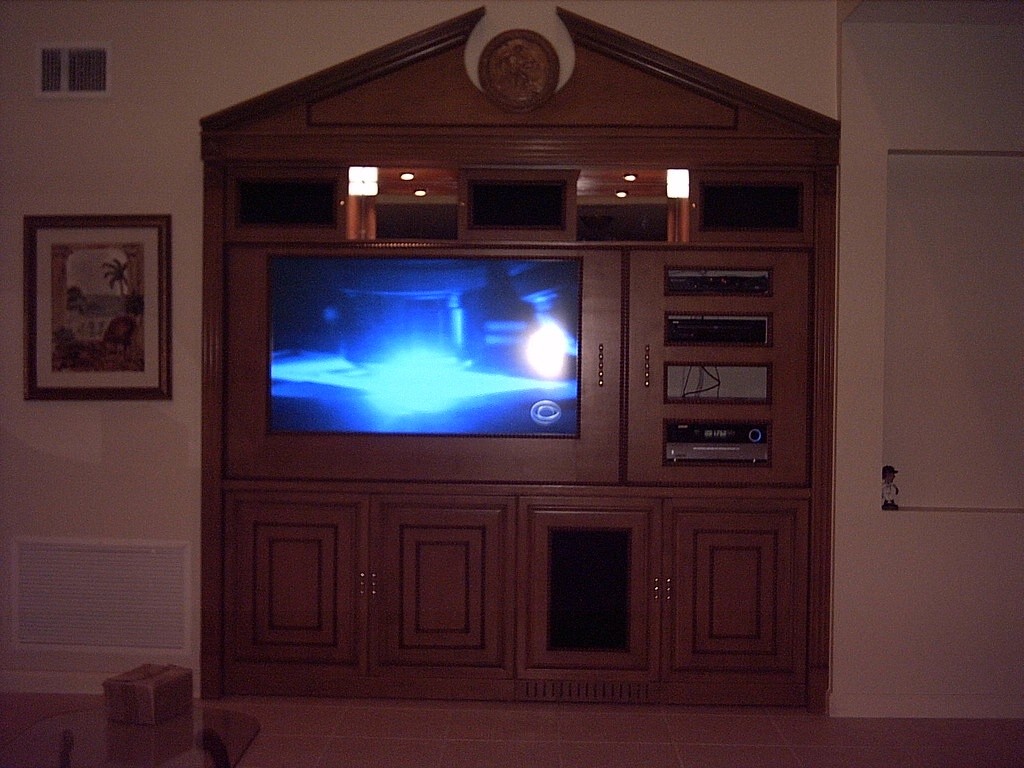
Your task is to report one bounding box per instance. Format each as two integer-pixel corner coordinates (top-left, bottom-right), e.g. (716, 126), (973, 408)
(265, 253), (582, 438)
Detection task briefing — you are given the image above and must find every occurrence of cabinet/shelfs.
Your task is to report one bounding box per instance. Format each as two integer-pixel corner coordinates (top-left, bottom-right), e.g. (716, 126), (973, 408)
(221, 480), (517, 698)
(624, 248), (807, 479)
(514, 494), (811, 705)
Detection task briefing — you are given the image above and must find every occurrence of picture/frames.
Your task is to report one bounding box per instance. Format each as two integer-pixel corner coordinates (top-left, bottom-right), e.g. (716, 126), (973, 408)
(21, 213), (174, 400)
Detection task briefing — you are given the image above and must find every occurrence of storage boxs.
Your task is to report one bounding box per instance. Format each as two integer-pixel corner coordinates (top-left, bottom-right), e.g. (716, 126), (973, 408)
(101, 662), (193, 722)
